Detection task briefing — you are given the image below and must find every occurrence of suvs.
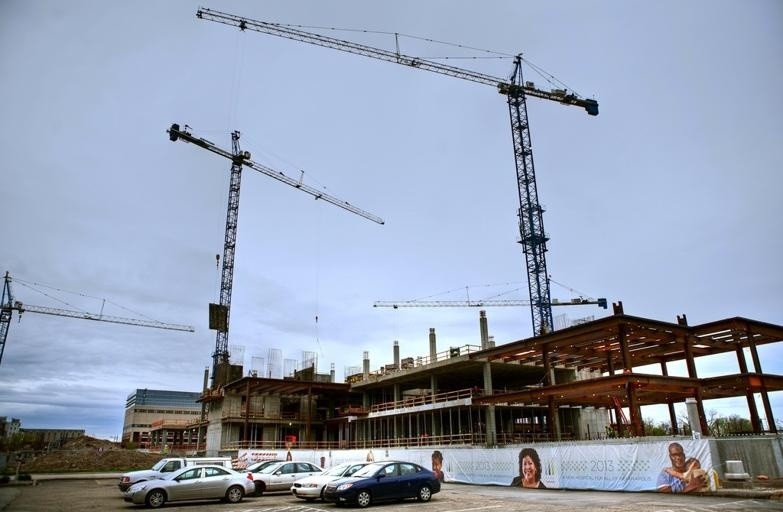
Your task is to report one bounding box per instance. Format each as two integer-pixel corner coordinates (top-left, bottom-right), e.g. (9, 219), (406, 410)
(119, 457), (232, 492)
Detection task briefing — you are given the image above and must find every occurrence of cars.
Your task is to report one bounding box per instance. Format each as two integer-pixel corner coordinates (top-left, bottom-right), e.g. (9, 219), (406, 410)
(124, 464), (256, 507)
(244, 459), (440, 507)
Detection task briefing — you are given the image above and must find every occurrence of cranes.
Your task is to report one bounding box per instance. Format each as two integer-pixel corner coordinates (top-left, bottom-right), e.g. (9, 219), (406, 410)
(193, 6), (601, 341)
(370, 275), (608, 334)
(0, 269), (196, 370)
(167, 120), (388, 372)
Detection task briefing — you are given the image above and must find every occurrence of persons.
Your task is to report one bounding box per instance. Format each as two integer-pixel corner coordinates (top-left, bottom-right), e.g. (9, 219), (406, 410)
(663, 459), (710, 494)
(429, 450), (445, 482)
(509, 448), (548, 489)
(657, 442), (706, 494)
